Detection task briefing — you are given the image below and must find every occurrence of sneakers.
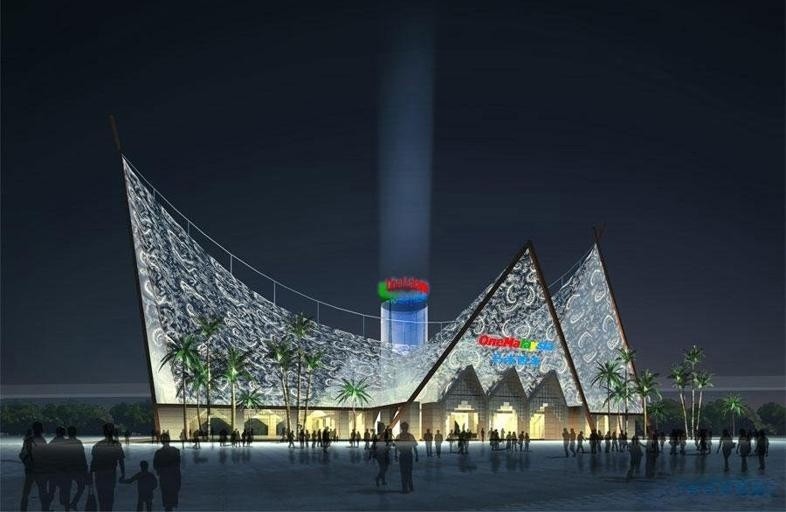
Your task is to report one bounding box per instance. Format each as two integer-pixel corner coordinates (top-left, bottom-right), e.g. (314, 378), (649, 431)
(65, 506), (70, 511)
(70, 503), (77, 510)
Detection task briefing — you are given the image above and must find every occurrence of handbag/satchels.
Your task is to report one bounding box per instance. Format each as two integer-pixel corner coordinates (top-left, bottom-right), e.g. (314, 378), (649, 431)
(86, 495), (98, 512)
